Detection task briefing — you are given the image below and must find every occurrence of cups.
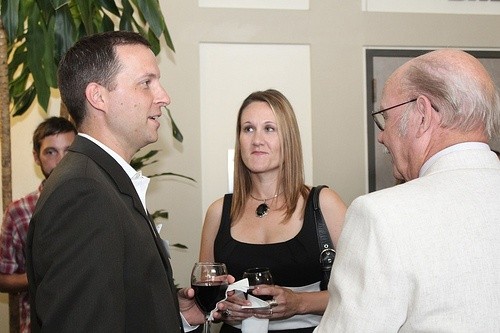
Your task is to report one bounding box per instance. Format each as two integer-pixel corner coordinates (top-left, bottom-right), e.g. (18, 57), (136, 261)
(243, 267), (274, 301)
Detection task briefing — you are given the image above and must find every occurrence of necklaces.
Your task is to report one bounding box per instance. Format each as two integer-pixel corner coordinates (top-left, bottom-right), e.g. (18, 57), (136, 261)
(247, 186), (289, 218)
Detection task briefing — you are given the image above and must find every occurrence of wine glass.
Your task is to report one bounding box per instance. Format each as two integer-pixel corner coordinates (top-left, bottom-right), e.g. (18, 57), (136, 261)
(190, 262), (228, 333)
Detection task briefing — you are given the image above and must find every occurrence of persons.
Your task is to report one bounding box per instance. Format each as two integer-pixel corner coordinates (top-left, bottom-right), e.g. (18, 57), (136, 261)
(24, 31), (237, 333)
(200, 89), (348, 333)
(0, 117), (79, 333)
(312, 48), (500, 333)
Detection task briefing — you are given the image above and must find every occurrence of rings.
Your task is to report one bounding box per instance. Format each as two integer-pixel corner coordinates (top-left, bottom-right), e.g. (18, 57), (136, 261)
(267, 299), (278, 307)
(270, 309), (272, 315)
(222, 308), (232, 317)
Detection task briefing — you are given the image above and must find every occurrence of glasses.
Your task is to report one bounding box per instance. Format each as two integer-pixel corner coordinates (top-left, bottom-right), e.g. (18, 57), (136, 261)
(372, 97), (438, 131)
